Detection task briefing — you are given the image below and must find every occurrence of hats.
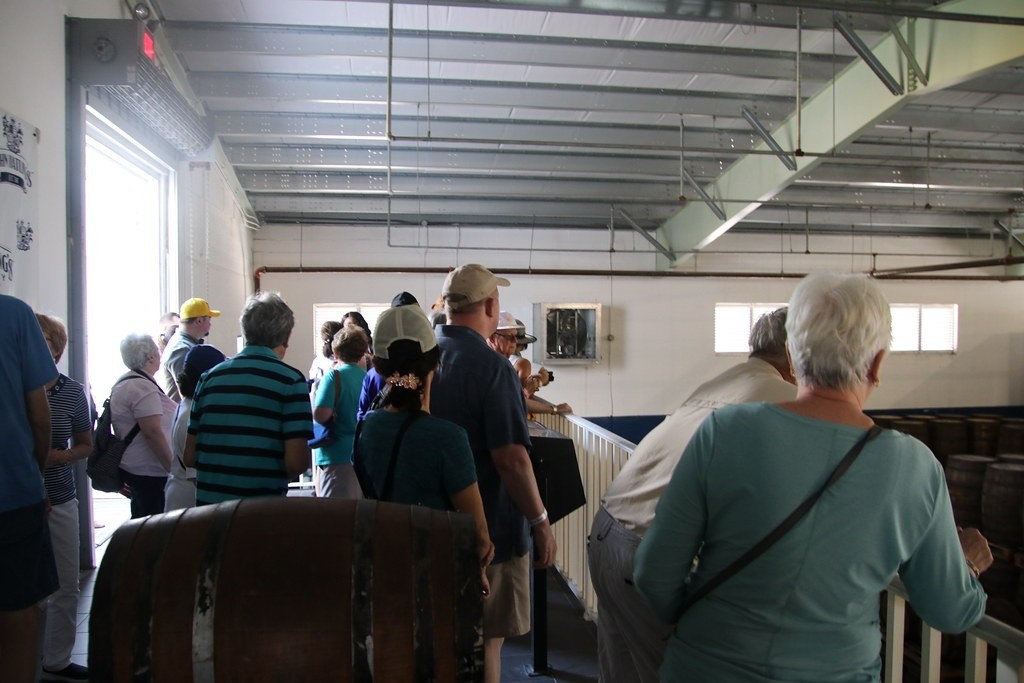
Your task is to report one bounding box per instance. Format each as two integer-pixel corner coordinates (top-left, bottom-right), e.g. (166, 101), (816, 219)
(371, 306), (438, 356)
(513, 318), (537, 344)
(181, 298), (222, 320)
(391, 293), (419, 307)
(441, 264), (512, 309)
(496, 311), (525, 329)
(184, 344), (226, 374)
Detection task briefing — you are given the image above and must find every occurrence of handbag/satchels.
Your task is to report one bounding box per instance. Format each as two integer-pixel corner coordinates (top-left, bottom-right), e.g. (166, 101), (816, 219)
(307, 369), (341, 448)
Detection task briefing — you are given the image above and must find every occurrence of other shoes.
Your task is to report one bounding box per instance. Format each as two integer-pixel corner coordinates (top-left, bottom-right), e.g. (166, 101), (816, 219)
(42, 662), (89, 682)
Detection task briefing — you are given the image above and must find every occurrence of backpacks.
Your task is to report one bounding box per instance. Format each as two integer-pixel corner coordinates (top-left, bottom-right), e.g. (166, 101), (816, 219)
(86, 375), (156, 493)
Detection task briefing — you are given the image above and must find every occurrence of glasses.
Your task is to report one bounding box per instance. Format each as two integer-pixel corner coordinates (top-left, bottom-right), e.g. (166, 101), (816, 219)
(494, 332), (518, 341)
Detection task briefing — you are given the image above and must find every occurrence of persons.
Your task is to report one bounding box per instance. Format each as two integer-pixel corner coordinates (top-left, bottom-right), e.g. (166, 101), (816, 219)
(32, 313), (93, 683)
(430, 263), (557, 682)
(163, 345), (226, 513)
(309, 311), (385, 498)
(183, 291), (316, 505)
(352, 305), (496, 599)
(490, 312), (574, 415)
(633, 265), (994, 683)
(159, 296), (220, 401)
(109, 332), (178, 519)
(391, 292), (418, 307)
(585, 305), (798, 683)
(0, 294), (60, 683)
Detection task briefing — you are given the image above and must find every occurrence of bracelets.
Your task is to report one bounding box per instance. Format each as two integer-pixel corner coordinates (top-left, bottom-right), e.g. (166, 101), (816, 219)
(530, 508), (548, 525)
(65, 449), (72, 466)
(551, 406), (557, 415)
(967, 559), (979, 577)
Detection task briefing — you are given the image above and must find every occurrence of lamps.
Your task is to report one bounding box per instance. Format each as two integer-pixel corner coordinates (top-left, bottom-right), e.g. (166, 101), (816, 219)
(132, 3), (159, 64)
